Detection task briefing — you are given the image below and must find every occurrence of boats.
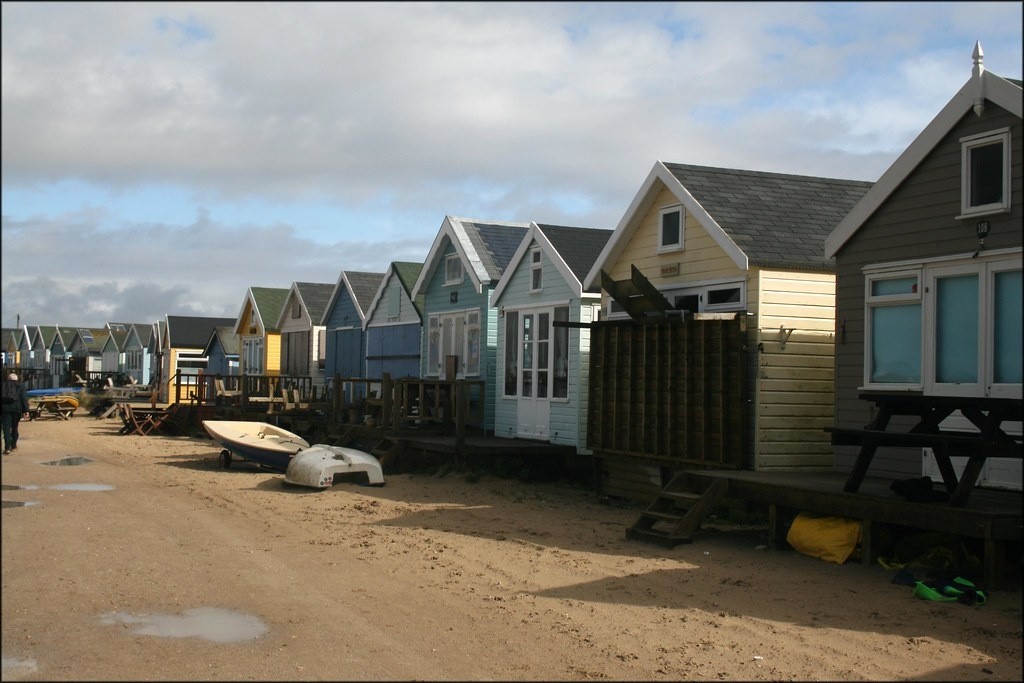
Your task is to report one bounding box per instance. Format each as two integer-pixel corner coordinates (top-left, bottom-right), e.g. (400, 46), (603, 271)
(202, 419), (386, 488)
(27, 395), (80, 414)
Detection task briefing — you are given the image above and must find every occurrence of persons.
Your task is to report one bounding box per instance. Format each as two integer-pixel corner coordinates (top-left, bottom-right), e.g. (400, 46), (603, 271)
(1, 373), (29, 454)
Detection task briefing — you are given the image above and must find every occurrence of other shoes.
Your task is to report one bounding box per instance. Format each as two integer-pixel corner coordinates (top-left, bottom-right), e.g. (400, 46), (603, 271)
(11, 448), (18, 453)
(4, 447), (10, 455)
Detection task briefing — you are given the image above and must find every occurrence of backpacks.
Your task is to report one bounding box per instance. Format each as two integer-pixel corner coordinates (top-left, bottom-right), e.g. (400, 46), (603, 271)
(1, 380), (19, 400)
